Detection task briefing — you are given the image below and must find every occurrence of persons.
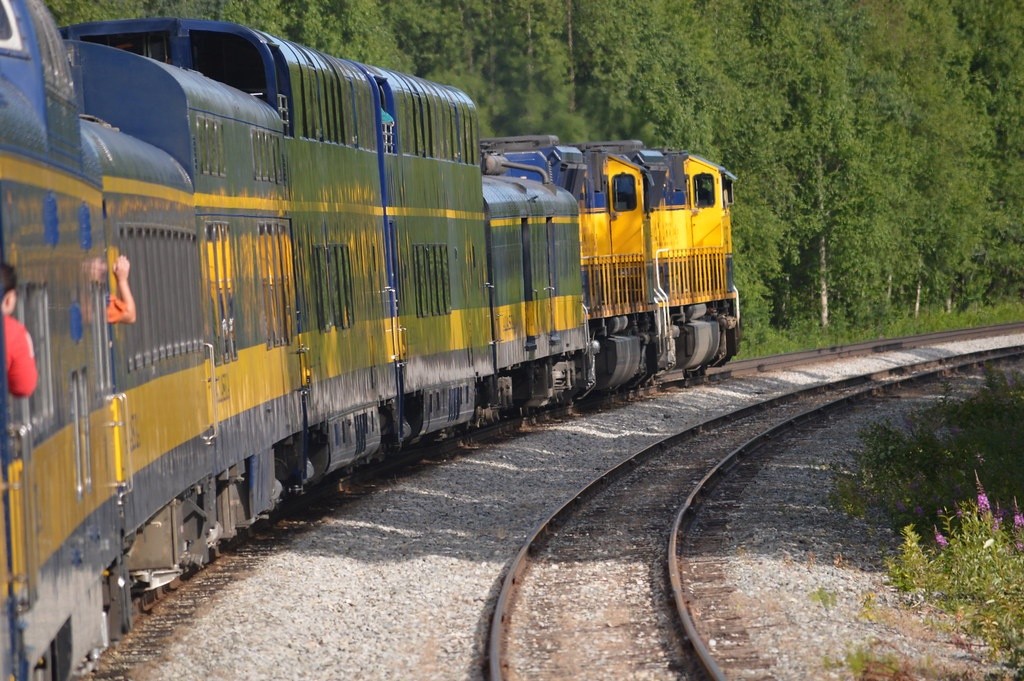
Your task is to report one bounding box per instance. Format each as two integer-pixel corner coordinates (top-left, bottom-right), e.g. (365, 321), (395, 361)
(106, 255), (137, 324)
(0, 262), (39, 399)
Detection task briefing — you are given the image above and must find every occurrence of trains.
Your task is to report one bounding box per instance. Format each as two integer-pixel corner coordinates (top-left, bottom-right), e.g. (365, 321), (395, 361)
(1, 1), (743, 681)
